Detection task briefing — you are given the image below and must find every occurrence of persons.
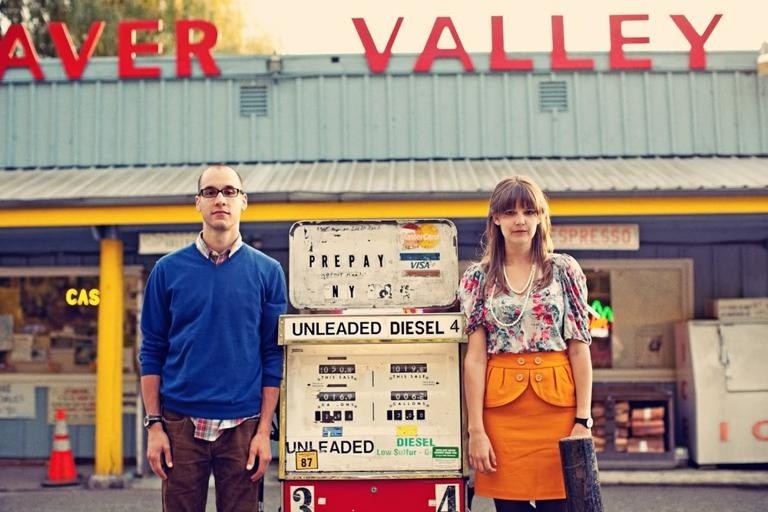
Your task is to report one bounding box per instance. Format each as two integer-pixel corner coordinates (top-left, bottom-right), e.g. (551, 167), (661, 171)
(138, 161), (289, 511)
(454, 174), (594, 511)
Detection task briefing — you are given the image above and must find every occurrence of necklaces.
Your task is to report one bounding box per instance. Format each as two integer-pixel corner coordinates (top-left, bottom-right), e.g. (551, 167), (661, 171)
(490, 249), (539, 327)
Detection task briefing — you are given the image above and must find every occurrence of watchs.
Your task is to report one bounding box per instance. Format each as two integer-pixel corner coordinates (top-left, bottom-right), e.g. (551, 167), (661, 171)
(574, 416), (594, 429)
(142, 416), (160, 429)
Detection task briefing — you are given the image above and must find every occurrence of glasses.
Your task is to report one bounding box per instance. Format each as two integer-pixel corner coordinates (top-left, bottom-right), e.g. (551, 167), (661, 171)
(197, 187), (245, 198)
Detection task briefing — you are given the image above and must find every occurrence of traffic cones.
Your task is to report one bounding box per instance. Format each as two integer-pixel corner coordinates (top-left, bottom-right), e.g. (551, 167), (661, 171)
(40, 408), (82, 486)
